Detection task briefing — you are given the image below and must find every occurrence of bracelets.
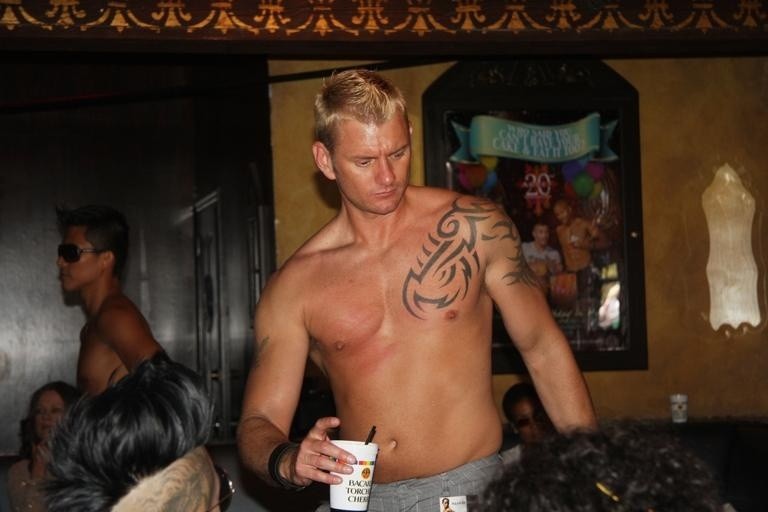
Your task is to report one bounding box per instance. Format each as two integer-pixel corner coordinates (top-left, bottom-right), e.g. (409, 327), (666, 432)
(270, 440), (310, 495)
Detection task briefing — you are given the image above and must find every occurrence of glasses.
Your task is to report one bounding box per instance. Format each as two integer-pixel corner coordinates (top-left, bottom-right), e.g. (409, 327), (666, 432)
(514, 408), (545, 428)
(58, 243), (97, 263)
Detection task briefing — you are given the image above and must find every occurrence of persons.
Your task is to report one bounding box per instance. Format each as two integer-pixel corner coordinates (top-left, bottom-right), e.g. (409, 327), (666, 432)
(232, 67), (604, 512)
(470, 415), (728, 511)
(521, 222), (564, 292)
(57, 203), (168, 400)
(34, 347), (237, 512)
(551, 196), (611, 288)
(4, 378), (81, 512)
(501, 380), (553, 477)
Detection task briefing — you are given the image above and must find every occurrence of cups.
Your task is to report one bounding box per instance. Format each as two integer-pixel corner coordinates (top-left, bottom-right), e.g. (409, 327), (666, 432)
(670, 394), (689, 424)
(326, 439), (379, 512)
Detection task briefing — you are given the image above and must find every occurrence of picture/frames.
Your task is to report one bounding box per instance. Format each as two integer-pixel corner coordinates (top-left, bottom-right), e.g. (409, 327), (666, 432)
(420, 59), (650, 375)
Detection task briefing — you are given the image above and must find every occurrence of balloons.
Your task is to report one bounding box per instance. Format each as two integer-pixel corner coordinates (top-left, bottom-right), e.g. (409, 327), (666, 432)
(460, 152), (508, 200)
(561, 158), (608, 202)
